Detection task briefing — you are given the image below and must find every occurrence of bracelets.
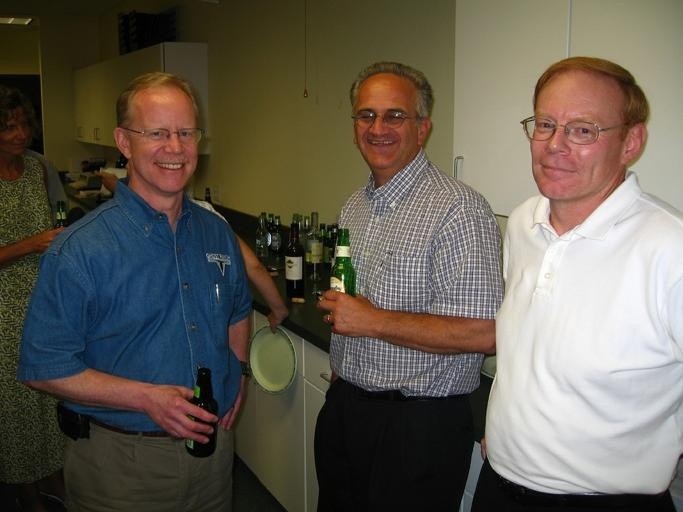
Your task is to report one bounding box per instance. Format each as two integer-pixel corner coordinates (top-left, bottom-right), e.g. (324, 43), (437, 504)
(237, 360), (251, 378)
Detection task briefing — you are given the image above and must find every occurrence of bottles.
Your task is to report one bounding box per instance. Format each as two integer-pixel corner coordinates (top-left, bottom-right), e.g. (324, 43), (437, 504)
(203, 187), (214, 205)
(184, 369), (220, 459)
(327, 228), (355, 297)
(116, 153), (128, 169)
(55, 202), (69, 230)
(255, 211), (282, 257)
(287, 211), (336, 297)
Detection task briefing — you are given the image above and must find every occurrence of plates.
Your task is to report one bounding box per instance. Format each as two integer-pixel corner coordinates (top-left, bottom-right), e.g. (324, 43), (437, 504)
(248, 322), (297, 396)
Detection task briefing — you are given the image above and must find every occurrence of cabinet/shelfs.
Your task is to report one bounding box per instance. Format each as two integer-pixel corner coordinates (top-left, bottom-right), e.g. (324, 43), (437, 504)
(75, 41), (210, 156)
(452, 0), (682, 219)
(303, 339), (333, 512)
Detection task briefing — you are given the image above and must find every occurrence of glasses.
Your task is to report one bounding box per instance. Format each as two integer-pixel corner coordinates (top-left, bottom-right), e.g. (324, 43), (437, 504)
(119, 127), (203, 144)
(351, 110), (420, 124)
(519, 116), (628, 145)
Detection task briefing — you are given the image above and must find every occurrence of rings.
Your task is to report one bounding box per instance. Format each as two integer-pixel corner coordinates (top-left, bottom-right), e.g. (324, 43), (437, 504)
(327, 315), (331, 324)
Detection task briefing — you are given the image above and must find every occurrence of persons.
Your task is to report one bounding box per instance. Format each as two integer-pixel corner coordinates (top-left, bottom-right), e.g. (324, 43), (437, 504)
(0, 86), (67, 511)
(189, 197), (289, 333)
(311, 58), (504, 512)
(94, 170), (121, 194)
(468, 55), (683, 512)
(15, 69), (253, 511)
